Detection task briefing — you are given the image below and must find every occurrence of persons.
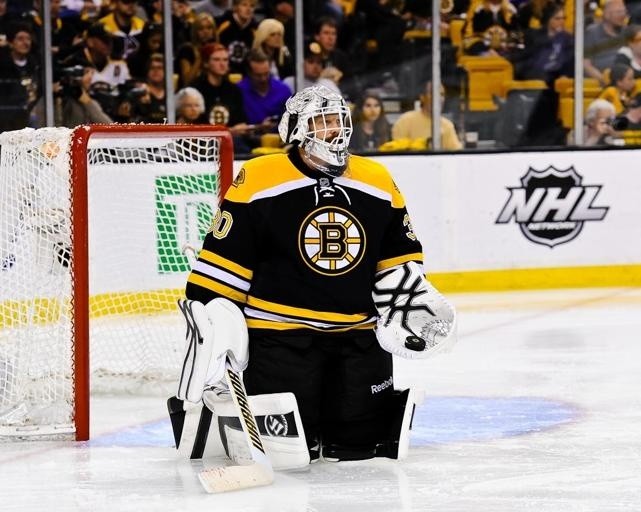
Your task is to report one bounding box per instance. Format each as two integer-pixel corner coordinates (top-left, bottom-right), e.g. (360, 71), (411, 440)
(0, 1), (640, 166)
(184, 82), (429, 465)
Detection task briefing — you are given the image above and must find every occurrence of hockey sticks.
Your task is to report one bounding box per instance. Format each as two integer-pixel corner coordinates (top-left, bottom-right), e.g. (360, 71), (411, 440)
(183, 245), (272, 492)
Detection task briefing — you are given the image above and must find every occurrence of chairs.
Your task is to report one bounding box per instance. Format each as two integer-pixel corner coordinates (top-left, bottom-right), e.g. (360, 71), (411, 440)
(231, 0), (641, 146)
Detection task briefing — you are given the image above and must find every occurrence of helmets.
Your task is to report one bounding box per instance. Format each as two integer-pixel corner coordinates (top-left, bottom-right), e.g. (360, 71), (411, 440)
(277, 84), (354, 180)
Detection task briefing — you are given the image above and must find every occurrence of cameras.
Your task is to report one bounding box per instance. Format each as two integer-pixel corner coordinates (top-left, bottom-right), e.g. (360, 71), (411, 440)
(606, 116), (629, 131)
(53, 64), (85, 100)
